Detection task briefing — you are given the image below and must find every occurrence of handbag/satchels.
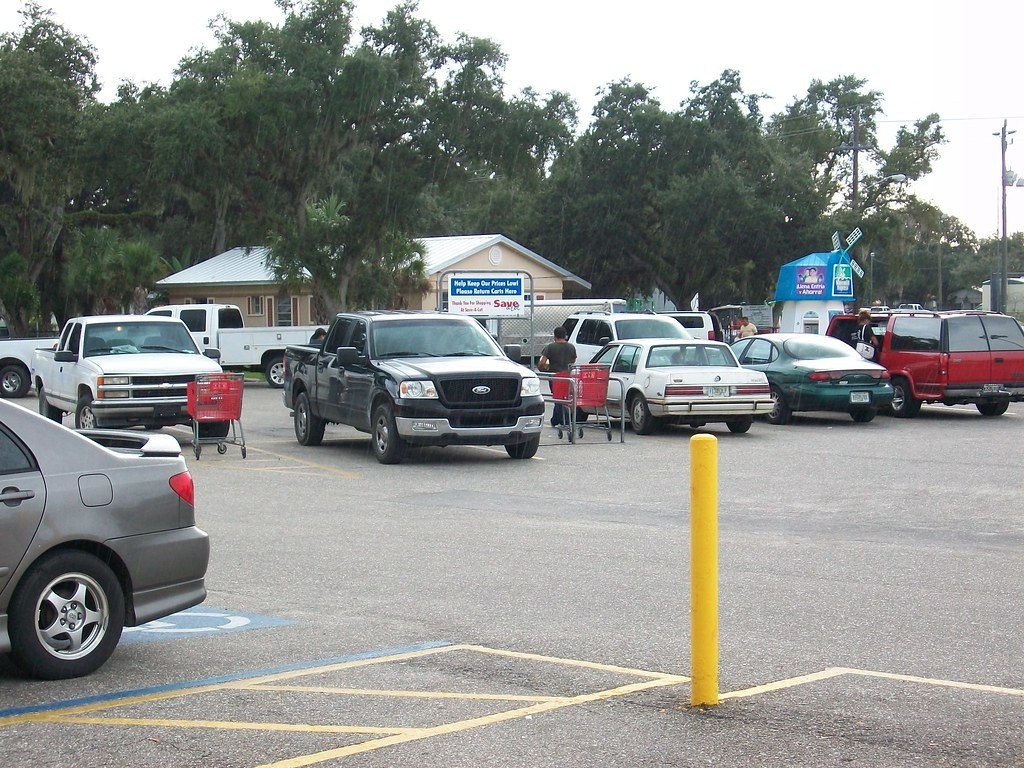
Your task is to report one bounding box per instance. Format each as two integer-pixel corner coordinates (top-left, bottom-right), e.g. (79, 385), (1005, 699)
(856, 340), (875, 359)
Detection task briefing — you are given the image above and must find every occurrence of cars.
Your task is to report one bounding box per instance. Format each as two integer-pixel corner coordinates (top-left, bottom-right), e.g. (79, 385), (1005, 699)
(729, 333), (896, 424)
(0, 396), (210, 677)
(869, 304), (923, 312)
(572, 337), (776, 436)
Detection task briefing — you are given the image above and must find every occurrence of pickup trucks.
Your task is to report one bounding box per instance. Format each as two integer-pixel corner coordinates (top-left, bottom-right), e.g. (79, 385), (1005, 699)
(32, 314), (230, 444)
(282, 309), (545, 464)
(143, 303), (334, 390)
(0, 318), (62, 399)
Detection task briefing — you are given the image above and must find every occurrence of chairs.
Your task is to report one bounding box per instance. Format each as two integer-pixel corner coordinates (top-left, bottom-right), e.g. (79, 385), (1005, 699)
(669, 351), (685, 367)
(144, 337), (165, 346)
(85, 336), (108, 351)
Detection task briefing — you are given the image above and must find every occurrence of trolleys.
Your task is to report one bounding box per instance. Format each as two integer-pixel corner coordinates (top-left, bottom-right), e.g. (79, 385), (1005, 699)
(186, 372), (247, 461)
(551, 362), (613, 444)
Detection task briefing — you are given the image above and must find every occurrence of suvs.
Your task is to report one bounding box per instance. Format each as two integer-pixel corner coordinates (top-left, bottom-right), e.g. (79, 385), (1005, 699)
(824, 308), (1024, 419)
(560, 309), (694, 423)
(655, 311), (725, 343)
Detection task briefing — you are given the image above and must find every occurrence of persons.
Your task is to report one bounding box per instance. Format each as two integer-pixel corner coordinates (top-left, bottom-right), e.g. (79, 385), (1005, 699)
(538, 327), (577, 426)
(737, 317), (758, 338)
(311, 328), (327, 341)
(848, 310), (879, 350)
(803, 267), (818, 283)
